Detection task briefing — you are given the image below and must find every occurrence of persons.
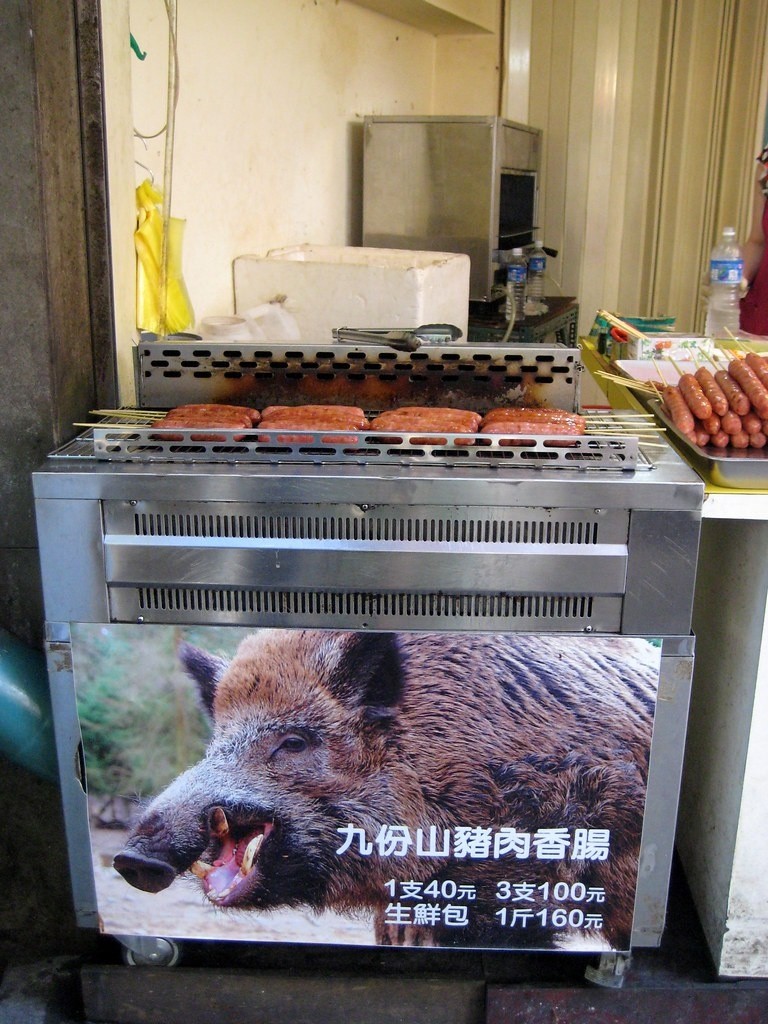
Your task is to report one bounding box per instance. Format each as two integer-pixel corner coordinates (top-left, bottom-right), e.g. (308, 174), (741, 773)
(700, 142), (768, 335)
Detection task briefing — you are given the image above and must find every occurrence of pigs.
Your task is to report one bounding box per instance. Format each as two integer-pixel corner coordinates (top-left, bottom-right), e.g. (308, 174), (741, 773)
(110, 625), (664, 950)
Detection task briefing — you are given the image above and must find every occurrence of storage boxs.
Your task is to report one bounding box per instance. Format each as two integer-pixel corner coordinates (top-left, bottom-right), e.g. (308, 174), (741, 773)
(232, 245), (471, 341)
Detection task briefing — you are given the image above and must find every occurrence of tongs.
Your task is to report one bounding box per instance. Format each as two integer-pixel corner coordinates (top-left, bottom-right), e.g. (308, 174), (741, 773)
(332, 323), (463, 353)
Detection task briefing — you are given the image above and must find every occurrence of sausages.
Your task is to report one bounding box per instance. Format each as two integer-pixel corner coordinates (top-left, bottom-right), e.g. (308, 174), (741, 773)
(150, 404), (587, 446)
(646, 352), (768, 451)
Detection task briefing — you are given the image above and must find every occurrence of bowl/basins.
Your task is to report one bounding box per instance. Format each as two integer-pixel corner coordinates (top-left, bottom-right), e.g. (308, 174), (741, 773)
(202, 315), (245, 336)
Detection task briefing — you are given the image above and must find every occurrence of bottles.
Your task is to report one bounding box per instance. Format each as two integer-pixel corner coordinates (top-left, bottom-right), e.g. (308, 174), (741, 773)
(525, 240), (548, 305)
(505, 247), (525, 321)
(704, 227), (745, 338)
